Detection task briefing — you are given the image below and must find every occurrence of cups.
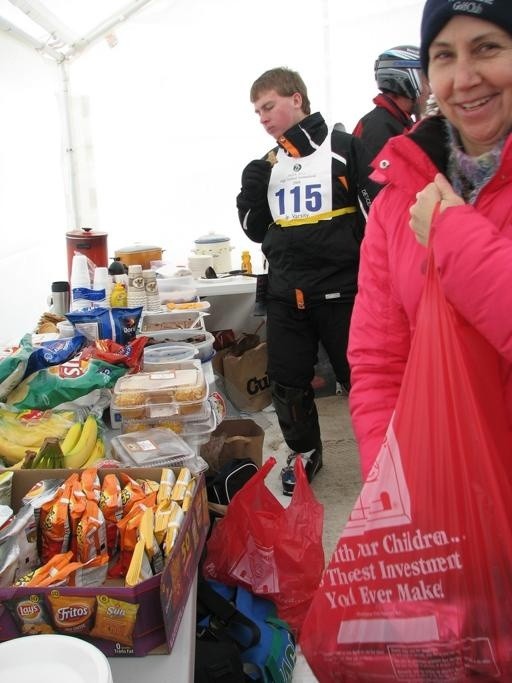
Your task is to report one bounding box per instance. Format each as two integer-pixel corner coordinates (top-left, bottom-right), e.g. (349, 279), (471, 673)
(126, 264), (164, 312)
(51, 281), (69, 314)
(69, 254), (91, 313)
(91, 265), (112, 308)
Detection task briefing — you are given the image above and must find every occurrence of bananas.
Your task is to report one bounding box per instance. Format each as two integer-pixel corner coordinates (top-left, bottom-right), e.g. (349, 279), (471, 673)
(0, 413), (106, 483)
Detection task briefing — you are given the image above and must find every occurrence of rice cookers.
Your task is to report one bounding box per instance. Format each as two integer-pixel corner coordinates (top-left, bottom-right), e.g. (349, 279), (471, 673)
(194, 234), (234, 274)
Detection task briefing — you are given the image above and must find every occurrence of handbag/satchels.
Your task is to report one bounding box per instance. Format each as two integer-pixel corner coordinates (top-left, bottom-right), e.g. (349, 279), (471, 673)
(194, 329), (301, 683)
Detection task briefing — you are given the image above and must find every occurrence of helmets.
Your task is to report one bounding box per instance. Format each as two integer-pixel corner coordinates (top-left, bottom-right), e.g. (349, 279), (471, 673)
(374, 46), (423, 98)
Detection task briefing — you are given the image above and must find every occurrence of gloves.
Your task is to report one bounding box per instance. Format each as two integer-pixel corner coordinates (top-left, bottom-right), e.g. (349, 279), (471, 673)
(237, 160), (271, 206)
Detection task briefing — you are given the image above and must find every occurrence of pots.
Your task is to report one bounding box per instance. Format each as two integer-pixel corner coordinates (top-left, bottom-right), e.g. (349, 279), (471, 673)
(111, 241), (167, 267)
(63, 225), (109, 275)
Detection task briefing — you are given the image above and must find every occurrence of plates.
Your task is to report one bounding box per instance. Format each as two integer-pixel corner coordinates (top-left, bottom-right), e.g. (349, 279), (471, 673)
(163, 299), (212, 312)
(198, 273), (231, 281)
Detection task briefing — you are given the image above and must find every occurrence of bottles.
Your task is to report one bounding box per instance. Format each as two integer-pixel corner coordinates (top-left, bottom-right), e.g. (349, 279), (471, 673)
(240, 249), (252, 275)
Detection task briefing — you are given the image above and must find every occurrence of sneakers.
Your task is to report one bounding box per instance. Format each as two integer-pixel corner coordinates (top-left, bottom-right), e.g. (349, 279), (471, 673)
(282, 448), (322, 495)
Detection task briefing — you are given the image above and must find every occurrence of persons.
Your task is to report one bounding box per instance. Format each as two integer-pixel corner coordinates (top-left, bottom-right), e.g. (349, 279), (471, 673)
(347, 0), (512, 485)
(347, 41), (433, 223)
(233, 65), (366, 496)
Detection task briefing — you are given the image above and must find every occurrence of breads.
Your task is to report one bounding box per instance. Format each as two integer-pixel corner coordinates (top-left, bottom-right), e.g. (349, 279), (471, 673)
(117, 382), (206, 436)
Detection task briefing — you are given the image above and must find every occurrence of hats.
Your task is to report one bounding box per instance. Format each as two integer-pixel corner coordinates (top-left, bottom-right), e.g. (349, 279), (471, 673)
(421, 0), (512, 78)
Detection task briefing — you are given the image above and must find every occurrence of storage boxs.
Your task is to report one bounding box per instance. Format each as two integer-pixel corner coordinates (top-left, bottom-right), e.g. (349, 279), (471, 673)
(0, 467), (211, 657)
(200, 418), (265, 470)
